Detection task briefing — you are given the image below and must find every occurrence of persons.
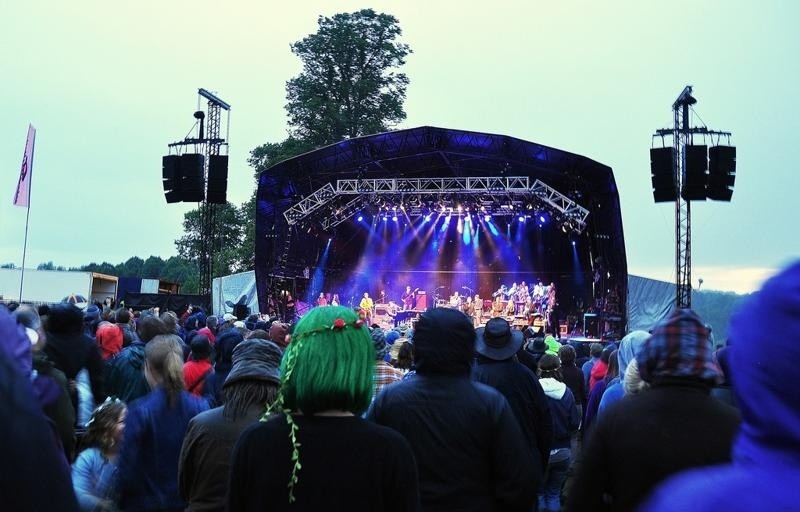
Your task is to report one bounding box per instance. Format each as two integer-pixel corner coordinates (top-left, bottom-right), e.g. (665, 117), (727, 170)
(582, 260), (799, 511)
(0, 301), (219, 512)
(178, 280), (584, 512)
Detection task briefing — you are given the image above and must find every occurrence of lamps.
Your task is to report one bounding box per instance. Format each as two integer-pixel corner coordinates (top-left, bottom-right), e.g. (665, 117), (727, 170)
(335, 198), (583, 232)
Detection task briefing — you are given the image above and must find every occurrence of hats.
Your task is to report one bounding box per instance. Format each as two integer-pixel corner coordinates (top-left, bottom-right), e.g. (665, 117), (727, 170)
(370, 323), (414, 360)
(222, 339), (282, 389)
(223, 313), (237, 322)
(474, 317), (524, 360)
(87, 304), (99, 312)
(528, 336), (549, 352)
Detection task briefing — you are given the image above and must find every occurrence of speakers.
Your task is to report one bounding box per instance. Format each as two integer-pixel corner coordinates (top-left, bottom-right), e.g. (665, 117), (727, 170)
(709, 145), (736, 202)
(682, 143), (707, 200)
(162, 155), (180, 203)
(584, 313), (599, 339)
(650, 147), (679, 203)
(179, 153), (205, 202)
(206, 154), (228, 203)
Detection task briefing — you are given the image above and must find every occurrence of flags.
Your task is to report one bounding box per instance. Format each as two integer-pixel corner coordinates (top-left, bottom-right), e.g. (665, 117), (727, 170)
(10, 122), (36, 210)
